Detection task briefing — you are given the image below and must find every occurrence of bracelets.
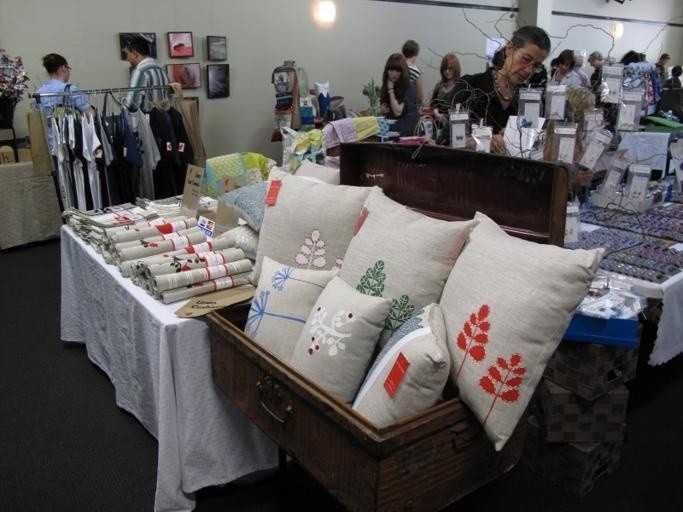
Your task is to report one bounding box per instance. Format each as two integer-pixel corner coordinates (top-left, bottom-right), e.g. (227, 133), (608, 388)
(386, 88), (394, 94)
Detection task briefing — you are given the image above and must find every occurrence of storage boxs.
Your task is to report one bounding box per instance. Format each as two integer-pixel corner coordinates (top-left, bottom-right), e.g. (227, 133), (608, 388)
(518, 315), (645, 508)
(206, 140), (569, 512)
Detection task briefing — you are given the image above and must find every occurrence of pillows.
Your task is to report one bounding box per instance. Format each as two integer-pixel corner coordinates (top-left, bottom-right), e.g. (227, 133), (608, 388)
(288, 275), (396, 405)
(338, 184), (480, 350)
(350, 302), (449, 430)
(439, 210), (607, 453)
(242, 255), (340, 366)
(248, 163), (383, 289)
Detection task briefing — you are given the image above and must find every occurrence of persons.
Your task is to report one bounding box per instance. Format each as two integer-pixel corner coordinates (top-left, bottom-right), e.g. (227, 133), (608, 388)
(173, 43), (192, 55)
(120, 35), (176, 112)
(36, 48), (97, 153)
(443, 24), (551, 120)
(370, 24), (683, 156)
(375, 52), (420, 137)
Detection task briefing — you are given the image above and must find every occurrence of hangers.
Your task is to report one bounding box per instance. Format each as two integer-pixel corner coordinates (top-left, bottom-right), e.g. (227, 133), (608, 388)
(48, 88), (185, 117)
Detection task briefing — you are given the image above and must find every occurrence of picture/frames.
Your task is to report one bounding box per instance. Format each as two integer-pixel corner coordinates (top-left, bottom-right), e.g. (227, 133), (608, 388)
(166, 31), (194, 58)
(165, 64), (201, 88)
(119, 32), (157, 60)
(206, 35), (226, 60)
(206, 63), (231, 98)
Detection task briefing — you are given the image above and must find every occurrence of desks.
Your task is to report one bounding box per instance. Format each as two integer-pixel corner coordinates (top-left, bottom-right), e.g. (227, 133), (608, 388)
(0, 161), (65, 250)
(564, 200), (682, 368)
(59, 194), (282, 510)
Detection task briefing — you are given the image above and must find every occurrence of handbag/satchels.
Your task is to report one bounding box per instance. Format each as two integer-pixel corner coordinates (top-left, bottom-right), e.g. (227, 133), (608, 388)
(27, 92), (55, 177)
(169, 83), (204, 167)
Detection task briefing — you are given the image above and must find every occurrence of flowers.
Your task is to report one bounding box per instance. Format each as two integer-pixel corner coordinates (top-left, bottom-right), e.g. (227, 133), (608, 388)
(0, 47), (30, 103)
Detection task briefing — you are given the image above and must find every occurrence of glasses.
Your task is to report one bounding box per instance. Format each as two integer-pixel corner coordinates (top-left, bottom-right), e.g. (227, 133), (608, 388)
(65, 65), (71, 70)
(442, 66), (453, 72)
(514, 46), (544, 74)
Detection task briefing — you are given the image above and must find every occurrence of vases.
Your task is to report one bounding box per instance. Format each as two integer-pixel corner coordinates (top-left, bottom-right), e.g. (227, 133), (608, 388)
(0, 97), (18, 130)
